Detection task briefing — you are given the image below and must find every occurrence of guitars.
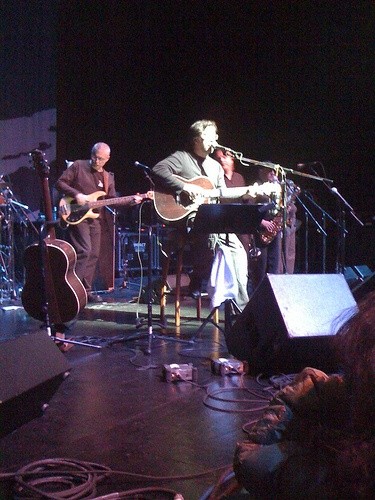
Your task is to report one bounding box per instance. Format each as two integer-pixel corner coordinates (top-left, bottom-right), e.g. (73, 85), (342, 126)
(58, 191), (154, 226)
(21, 149), (87, 325)
(153, 174), (282, 221)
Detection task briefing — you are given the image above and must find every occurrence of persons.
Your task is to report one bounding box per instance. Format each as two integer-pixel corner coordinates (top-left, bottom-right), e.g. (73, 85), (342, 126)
(233, 291), (375, 500)
(257, 159), (301, 273)
(214, 146), (274, 259)
(149, 121), (265, 298)
(55, 142), (143, 302)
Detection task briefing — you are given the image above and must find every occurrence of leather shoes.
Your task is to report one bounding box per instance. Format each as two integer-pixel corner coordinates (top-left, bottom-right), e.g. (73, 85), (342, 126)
(87, 293), (103, 302)
(189, 280), (201, 300)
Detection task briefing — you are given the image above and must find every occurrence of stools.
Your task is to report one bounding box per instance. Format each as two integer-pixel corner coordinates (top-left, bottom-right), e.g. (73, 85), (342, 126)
(159, 233), (219, 328)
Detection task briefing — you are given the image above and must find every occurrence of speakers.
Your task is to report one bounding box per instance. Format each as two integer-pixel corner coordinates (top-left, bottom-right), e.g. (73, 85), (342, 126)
(0, 329), (71, 438)
(151, 234), (169, 270)
(116, 232), (157, 271)
(226, 266), (375, 373)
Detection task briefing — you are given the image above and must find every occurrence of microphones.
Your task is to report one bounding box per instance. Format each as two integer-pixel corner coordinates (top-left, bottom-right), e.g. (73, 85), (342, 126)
(290, 182), (300, 191)
(297, 161), (318, 168)
(211, 142), (237, 155)
(8, 199), (29, 210)
(135, 161), (149, 169)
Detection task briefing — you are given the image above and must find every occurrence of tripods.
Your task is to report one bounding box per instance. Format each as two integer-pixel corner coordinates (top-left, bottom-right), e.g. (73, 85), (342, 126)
(0, 207), (24, 300)
(107, 202), (269, 354)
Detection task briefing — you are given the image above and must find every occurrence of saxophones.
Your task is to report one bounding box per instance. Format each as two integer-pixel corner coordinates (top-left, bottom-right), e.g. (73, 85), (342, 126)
(260, 182), (301, 246)
